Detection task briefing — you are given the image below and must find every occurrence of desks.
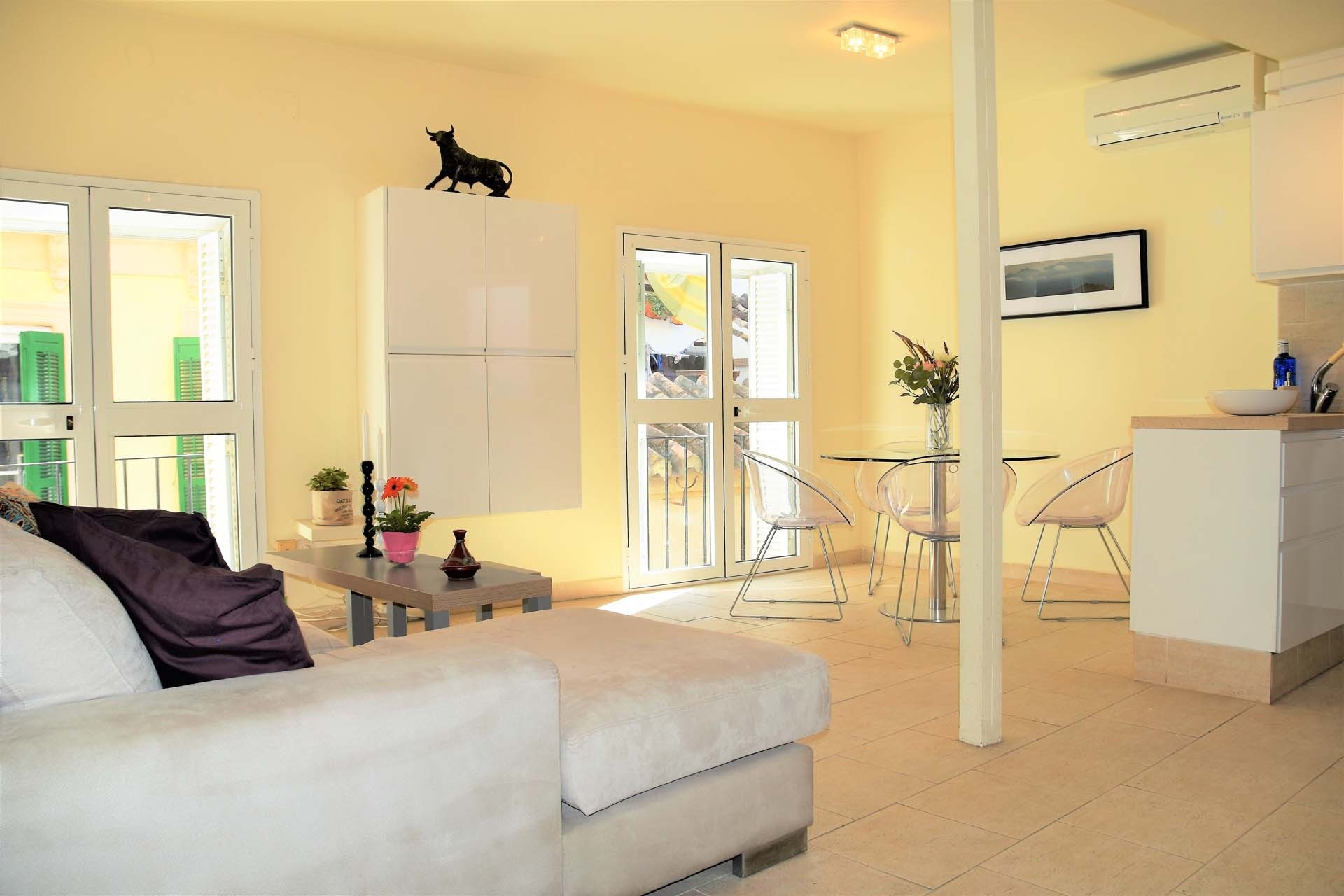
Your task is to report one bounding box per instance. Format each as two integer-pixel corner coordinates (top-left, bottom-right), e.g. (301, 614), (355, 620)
(266, 544), (552, 647)
(818, 450), (1061, 623)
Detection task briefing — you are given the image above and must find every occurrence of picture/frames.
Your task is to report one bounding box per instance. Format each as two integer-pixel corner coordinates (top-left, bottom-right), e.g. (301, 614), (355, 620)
(1001, 229), (1149, 320)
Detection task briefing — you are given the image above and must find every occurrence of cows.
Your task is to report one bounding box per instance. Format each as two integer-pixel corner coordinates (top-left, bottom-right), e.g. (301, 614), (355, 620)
(424, 122), (513, 199)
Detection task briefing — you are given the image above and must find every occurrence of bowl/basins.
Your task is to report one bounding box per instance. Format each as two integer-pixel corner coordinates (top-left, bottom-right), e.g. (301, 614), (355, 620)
(1209, 390), (1298, 415)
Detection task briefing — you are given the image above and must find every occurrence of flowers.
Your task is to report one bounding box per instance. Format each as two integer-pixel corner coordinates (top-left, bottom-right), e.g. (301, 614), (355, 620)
(889, 330), (960, 404)
(369, 476), (435, 534)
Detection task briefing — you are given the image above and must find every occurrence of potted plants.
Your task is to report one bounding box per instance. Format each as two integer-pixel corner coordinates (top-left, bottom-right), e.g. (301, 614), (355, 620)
(306, 466), (354, 526)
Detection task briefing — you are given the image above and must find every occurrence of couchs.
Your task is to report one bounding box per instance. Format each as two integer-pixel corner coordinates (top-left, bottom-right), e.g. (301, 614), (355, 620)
(0, 484), (830, 896)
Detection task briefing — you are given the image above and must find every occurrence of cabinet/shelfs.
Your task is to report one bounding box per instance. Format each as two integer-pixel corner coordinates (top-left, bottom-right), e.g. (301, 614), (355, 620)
(366, 187), (582, 519)
(1129, 413), (1344, 706)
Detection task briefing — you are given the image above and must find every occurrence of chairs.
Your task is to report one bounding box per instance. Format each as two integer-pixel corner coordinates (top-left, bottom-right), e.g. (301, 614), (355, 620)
(730, 441), (1134, 646)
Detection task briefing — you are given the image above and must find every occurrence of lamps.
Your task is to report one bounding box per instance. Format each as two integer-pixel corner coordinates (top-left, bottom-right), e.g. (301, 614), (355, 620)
(836, 24), (898, 59)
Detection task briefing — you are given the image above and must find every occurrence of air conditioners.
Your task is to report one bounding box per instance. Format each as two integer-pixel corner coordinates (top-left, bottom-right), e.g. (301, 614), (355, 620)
(1083, 52), (1267, 154)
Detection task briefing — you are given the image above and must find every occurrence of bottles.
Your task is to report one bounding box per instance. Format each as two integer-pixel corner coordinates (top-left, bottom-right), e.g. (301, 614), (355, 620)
(439, 530), (481, 581)
(1273, 341), (1296, 389)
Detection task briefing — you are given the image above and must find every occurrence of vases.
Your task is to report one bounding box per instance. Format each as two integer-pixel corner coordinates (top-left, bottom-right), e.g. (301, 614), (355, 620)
(926, 404), (954, 451)
(382, 532), (419, 568)
